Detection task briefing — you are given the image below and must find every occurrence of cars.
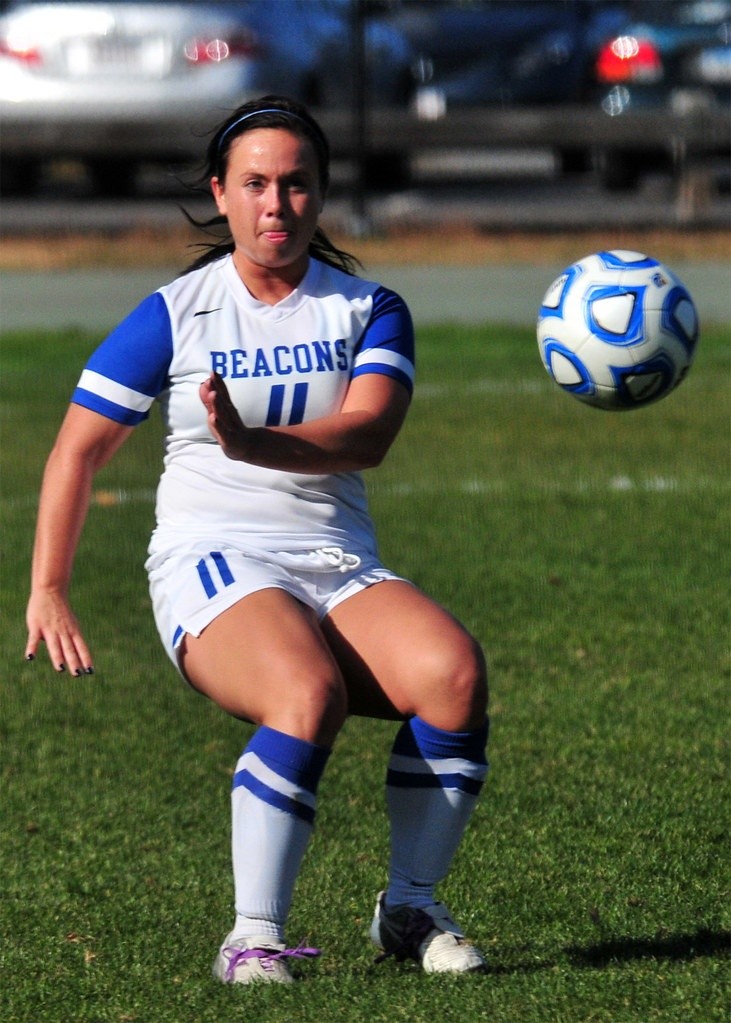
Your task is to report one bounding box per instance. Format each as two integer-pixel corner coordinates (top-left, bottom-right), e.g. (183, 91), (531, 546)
(2, 1), (273, 194)
(303, 0), (675, 196)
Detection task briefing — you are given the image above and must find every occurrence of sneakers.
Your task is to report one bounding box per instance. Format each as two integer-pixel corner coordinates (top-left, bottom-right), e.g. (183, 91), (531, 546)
(372, 892), (485, 976)
(212, 937), (296, 986)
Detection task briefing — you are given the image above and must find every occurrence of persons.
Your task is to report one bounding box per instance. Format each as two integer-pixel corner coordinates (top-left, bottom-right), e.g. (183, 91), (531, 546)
(25, 93), (496, 986)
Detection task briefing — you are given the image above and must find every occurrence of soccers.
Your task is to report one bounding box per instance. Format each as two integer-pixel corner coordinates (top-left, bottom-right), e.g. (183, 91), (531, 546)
(536, 250), (699, 411)
